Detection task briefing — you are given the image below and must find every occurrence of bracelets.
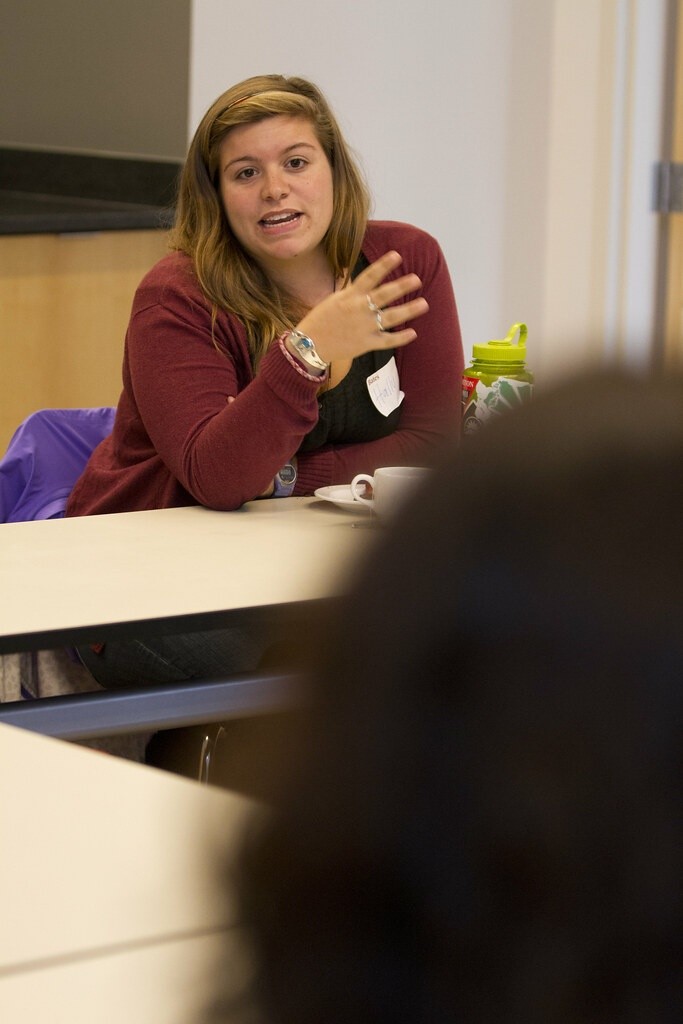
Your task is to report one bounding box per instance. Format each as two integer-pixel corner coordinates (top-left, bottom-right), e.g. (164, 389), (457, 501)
(278, 328), (328, 383)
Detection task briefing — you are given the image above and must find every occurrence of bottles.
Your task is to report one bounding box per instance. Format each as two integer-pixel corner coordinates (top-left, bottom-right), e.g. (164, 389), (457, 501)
(460, 322), (534, 443)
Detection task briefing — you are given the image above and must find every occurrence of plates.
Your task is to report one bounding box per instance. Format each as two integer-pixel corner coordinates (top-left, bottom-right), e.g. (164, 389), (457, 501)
(315, 483), (372, 513)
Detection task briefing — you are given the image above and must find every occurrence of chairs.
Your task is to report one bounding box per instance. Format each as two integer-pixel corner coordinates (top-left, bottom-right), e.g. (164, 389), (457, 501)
(11, 410), (235, 789)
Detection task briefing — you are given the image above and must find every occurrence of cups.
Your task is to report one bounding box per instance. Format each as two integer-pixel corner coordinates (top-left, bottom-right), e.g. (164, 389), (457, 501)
(350, 466), (431, 520)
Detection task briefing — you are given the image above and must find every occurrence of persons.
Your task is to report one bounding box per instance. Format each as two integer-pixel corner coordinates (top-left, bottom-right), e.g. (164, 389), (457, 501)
(59, 75), (464, 785)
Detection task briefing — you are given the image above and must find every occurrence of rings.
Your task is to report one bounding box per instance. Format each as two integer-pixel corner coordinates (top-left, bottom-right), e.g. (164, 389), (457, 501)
(366, 294), (384, 333)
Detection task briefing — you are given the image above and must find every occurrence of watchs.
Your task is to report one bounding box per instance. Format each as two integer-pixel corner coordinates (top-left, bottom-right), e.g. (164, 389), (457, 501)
(272, 460), (297, 499)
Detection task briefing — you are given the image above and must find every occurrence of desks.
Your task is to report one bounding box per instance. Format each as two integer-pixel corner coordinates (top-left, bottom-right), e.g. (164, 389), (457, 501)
(0, 496), (382, 743)
(0, 722), (273, 1024)
